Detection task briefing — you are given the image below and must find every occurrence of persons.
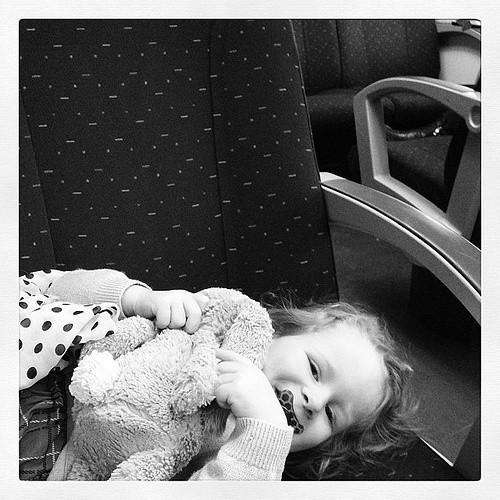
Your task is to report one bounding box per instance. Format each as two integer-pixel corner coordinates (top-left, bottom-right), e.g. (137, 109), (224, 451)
(19, 269), (416, 480)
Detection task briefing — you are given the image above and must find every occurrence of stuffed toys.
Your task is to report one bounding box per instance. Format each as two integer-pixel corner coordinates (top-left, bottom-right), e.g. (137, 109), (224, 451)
(62, 288), (275, 480)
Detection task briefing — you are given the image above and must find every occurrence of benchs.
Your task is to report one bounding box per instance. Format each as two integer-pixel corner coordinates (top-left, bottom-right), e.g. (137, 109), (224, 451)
(19, 18), (481, 482)
(293, 18), (481, 356)
